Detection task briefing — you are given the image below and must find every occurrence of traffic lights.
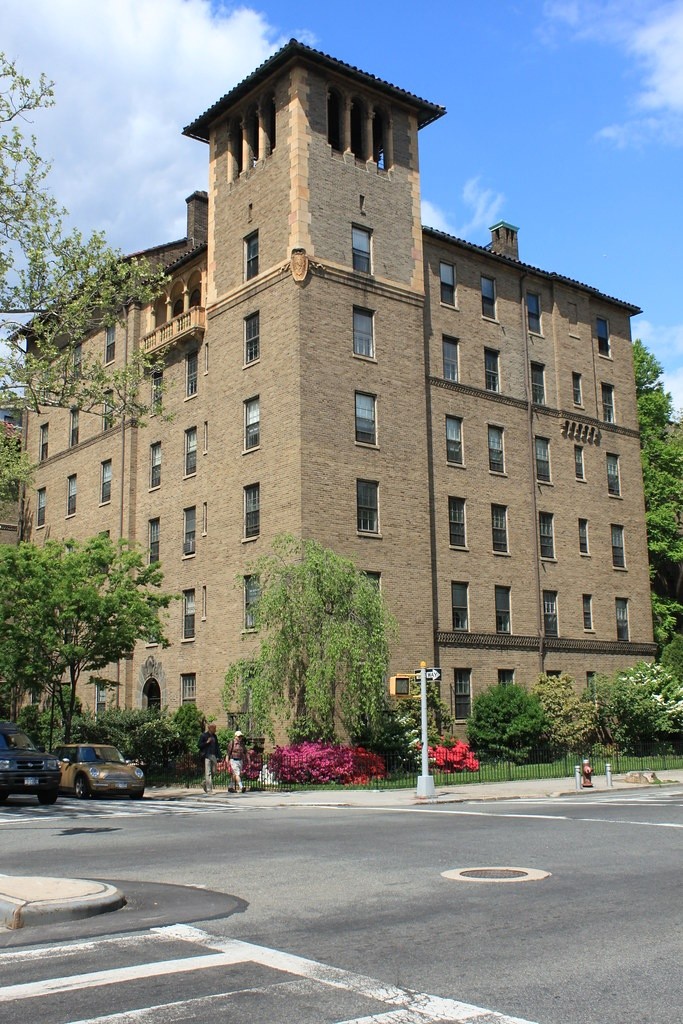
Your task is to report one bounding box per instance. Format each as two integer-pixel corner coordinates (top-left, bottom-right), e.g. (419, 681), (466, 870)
(389, 676), (411, 698)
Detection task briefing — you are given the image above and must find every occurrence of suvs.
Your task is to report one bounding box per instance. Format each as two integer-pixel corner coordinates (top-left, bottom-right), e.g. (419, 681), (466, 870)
(0, 719), (63, 805)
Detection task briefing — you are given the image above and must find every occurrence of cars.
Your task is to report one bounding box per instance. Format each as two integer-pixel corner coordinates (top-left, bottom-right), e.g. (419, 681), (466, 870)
(52, 742), (146, 800)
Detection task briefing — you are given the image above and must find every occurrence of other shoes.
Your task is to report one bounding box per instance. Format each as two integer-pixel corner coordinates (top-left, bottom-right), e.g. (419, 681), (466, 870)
(207, 791), (217, 795)
(242, 787), (246, 793)
(200, 781), (207, 793)
(228, 788), (237, 793)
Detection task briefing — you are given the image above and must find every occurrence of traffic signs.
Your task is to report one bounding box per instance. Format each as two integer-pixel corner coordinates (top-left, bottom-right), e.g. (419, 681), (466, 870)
(414, 668), (442, 682)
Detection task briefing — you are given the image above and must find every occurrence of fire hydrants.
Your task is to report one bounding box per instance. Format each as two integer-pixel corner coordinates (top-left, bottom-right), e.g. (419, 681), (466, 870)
(582, 756), (593, 787)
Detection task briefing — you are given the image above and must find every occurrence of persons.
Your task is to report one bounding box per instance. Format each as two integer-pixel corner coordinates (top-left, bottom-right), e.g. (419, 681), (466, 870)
(198, 723), (222, 795)
(225, 730), (247, 793)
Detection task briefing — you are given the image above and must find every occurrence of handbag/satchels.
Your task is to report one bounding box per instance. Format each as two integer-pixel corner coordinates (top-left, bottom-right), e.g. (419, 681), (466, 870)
(223, 739), (234, 761)
(198, 733), (209, 758)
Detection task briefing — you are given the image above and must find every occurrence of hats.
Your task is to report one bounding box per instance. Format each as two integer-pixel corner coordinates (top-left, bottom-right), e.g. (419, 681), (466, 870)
(235, 731), (244, 737)
